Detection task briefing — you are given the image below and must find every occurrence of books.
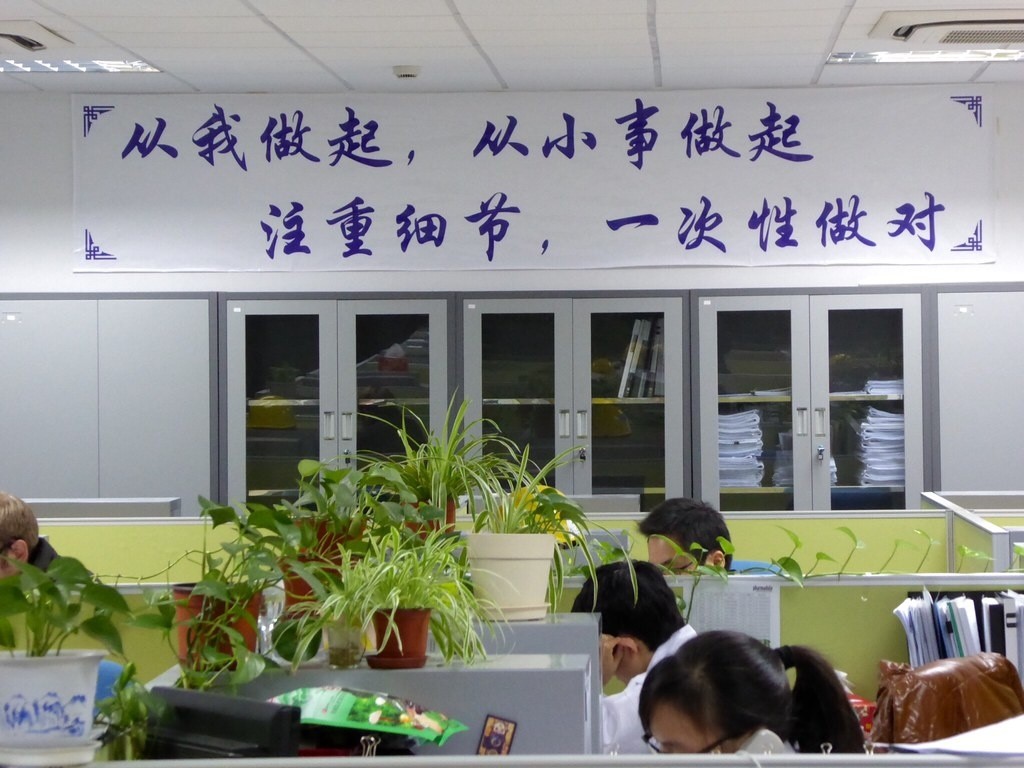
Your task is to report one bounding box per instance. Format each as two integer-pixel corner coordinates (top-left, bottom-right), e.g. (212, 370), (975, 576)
(617, 318), (665, 398)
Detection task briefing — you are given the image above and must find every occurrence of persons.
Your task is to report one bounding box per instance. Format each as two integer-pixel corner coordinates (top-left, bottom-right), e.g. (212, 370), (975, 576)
(571, 560), (698, 756)
(639, 630), (867, 754)
(0, 493), (106, 586)
(639, 497), (733, 575)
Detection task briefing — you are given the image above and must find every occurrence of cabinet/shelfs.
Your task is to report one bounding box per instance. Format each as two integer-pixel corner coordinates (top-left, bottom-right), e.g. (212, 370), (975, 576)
(0, 289), (1023, 508)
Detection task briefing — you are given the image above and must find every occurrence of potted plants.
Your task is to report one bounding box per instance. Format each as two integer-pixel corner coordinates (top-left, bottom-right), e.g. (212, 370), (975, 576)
(0, 387), (1024, 766)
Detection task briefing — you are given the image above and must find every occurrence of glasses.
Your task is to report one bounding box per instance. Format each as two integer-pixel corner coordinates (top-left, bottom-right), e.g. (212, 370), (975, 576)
(661, 561), (692, 576)
(641, 731), (748, 754)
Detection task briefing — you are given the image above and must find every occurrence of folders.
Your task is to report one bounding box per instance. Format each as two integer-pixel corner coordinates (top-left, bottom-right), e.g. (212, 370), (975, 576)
(996, 593), (1019, 672)
